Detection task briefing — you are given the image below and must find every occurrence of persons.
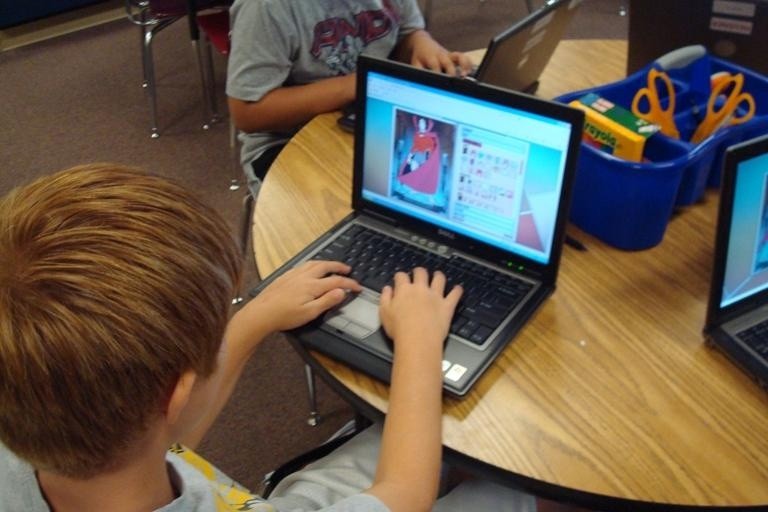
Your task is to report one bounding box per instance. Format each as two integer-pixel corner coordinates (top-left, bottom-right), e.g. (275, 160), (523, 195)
(397, 117), (440, 194)
(225, 0), (471, 202)
(0, 162), (582, 512)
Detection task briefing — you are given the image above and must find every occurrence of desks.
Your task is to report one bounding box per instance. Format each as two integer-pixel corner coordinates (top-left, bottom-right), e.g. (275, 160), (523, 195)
(248, 41), (768, 510)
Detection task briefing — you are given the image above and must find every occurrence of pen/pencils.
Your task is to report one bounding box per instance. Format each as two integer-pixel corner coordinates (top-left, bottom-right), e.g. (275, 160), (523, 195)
(564, 234), (587, 252)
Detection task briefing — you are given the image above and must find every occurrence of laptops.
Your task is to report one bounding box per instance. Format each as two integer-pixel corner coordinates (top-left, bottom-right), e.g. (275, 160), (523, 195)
(336, 0), (570, 131)
(248, 53), (585, 400)
(703, 135), (768, 397)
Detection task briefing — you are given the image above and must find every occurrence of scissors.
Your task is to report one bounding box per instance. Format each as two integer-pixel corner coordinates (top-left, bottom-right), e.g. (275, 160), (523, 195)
(631, 68), (755, 144)
